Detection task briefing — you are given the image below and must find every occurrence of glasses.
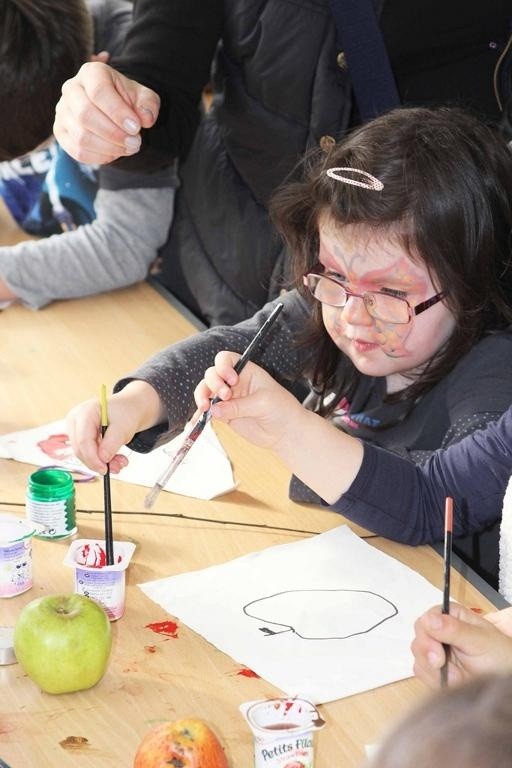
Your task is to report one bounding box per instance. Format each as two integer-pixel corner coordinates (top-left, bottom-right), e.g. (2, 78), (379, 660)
(304, 272), (447, 325)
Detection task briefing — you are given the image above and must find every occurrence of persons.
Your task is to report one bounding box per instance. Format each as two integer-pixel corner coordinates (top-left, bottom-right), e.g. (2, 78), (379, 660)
(196, 351), (512, 607)
(63, 106), (511, 510)
(370, 603), (512, 768)
(1, 0), (205, 310)
(52, 0), (511, 330)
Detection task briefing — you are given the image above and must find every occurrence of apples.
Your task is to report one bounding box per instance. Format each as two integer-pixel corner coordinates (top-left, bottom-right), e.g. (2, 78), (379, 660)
(133, 718), (229, 768)
(13, 593), (112, 695)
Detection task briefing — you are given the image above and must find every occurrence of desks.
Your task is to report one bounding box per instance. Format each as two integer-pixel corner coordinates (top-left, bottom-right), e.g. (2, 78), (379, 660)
(0, 191), (512, 768)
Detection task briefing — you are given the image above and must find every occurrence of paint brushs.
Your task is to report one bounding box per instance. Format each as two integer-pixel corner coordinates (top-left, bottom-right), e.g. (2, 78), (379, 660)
(100, 385), (114, 566)
(440, 498), (452, 694)
(142, 302), (284, 509)
